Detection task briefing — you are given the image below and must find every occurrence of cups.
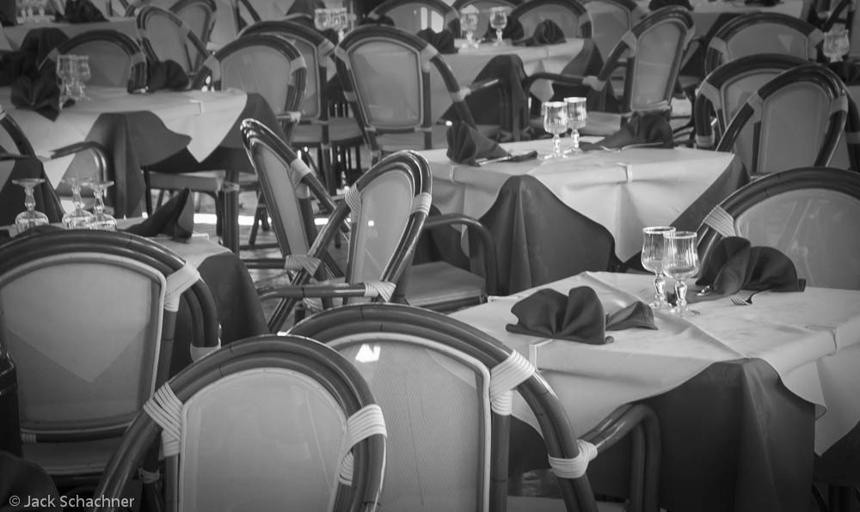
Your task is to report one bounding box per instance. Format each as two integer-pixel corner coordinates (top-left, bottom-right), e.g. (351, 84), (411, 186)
(333, 8), (348, 40)
(564, 97), (587, 157)
(313, 9), (331, 31)
(60, 177), (94, 229)
(86, 181), (118, 230)
(641, 226), (676, 313)
(57, 54), (77, 100)
(12, 179), (49, 234)
(460, 7), (478, 53)
(543, 101), (568, 160)
(489, 7), (507, 48)
(823, 29), (849, 62)
(67, 54), (86, 92)
(662, 232), (700, 317)
(70, 56), (90, 99)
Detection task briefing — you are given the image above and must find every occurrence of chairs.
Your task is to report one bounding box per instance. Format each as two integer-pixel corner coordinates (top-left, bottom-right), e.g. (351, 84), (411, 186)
(0, 229), (221, 512)
(817, 0), (854, 82)
(716, 62), (852, 183)
(237, 18), (365, 248)
(286, 300), (663, 512)
(451, 0), (518, 38)
(83, 332), (388, 512)
(136, 6), (211, 90)
(169, 0), (217, 72)
(0, 106), (115, 224)
(581, 0), (632, 65)
(510, 0), (592, 41)
(695, 52), (860, 172)
(268, 147), (433, 334)
(238, 117), (498, 327)
(334, 23), (478, 170)
(64, 28), (147, 89)
(367, 0), (462, 40)
(522, 4), (695, 138)
(694, 164), (860, 291)
(143, 33), (308, 245)
(703, 11), (825, 79)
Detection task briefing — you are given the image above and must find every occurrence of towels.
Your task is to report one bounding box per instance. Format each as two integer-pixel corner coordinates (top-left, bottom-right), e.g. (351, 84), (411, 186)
(742, 246), (806, 292)
(151, 59), (189, 90)
(607, 302), (657, 329)
(125, 188), (194, 241)
(487, 16), (524, 41)
(417, 28), (454, 49)
(506, 286), (613, 346)
(642, 113), (673, 149)
(11, 75), (60, 110)
(579, 110), (644, 151)
(446, 121), (509, 161)
(696, 236), (751, 297)
(65, 0), (105, 23)
(526, 19), (565, 46)
